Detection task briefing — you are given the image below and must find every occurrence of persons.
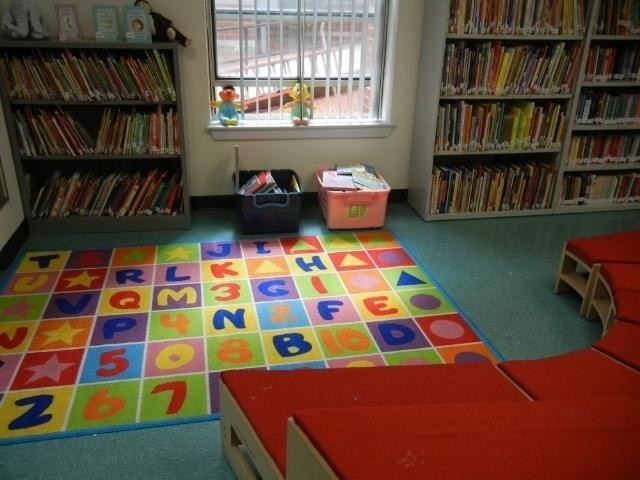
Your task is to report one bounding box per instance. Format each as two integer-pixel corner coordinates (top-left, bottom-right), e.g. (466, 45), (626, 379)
(284, 81), (320, 125)
(210, 84), (248, 126)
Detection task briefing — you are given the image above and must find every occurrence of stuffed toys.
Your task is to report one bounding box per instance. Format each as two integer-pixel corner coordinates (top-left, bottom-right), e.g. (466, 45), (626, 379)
(1, 0), (50, 40)
(134, 0), (194, 48)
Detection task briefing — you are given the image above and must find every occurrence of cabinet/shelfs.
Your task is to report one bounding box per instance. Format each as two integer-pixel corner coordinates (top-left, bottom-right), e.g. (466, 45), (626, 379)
(1, 39), (194, 235)
(550, 0), (638, 215)
(406, 0), (596, 222)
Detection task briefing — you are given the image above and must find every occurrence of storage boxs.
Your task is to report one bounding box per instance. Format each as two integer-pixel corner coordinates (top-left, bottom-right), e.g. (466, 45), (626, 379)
(231, 168), (304, 234)
(315, 163), (390, 229)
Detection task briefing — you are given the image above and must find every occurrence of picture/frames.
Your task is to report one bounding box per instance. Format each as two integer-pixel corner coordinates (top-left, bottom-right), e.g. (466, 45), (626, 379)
(56, 4), (84, 42)
(123, 6), (153, 44)
(92, 4), (122, 44)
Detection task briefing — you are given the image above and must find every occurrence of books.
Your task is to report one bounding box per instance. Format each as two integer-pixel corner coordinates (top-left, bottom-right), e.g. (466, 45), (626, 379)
(320, 160), (385, 193)
(238, 168), (301, 195)
(2, 48), (184, 220)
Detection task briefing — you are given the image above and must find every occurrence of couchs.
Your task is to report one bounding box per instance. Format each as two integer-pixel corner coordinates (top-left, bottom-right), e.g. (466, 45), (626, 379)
(218, 227), (640, 479)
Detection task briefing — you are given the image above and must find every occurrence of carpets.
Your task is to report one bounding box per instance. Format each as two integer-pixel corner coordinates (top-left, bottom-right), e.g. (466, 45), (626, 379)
(2, 228), (515, 449)
